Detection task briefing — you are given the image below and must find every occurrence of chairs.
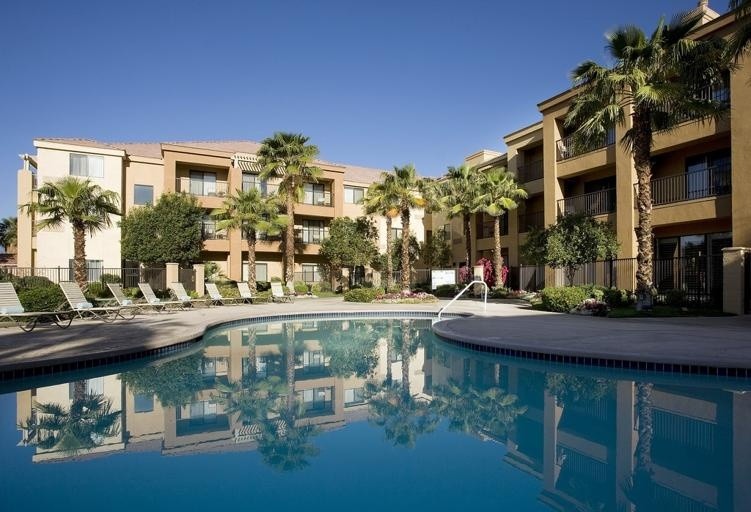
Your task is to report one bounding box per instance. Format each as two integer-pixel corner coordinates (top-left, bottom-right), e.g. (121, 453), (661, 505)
(0, 282), (294, 332)
